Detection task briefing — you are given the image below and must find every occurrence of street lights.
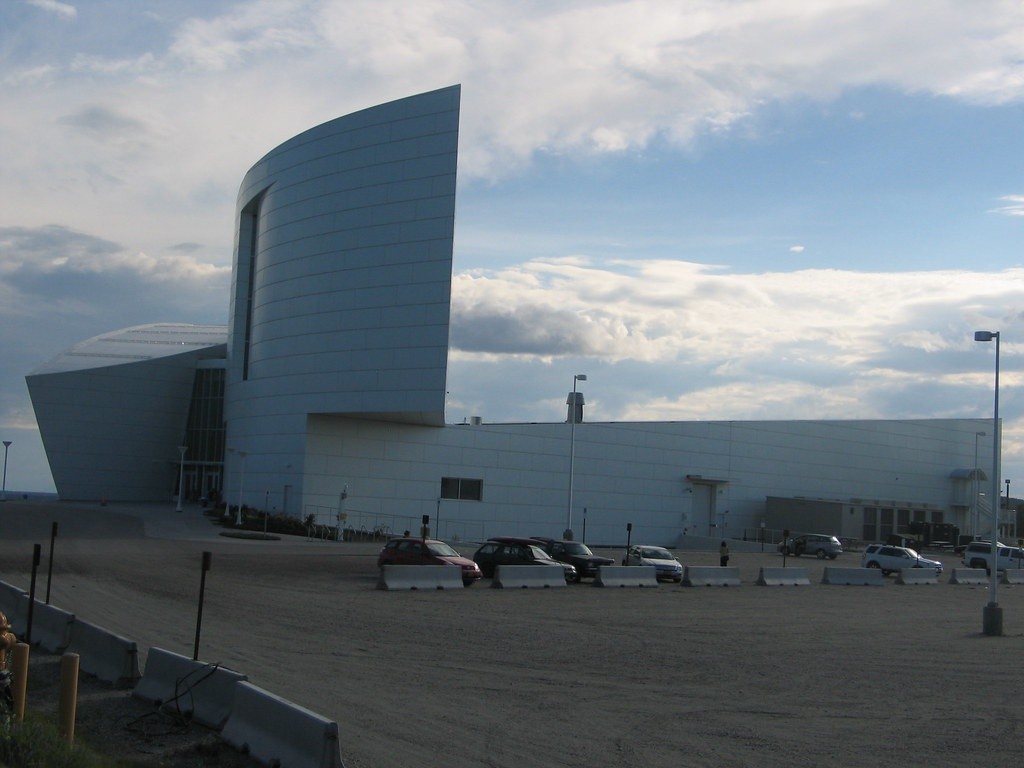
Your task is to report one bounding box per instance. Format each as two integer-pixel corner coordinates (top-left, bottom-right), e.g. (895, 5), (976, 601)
(974, 330), (1005, 635)
(562, 374), (587, 544)
(972, 431), (987, 541)
(0, 441), (13, 500)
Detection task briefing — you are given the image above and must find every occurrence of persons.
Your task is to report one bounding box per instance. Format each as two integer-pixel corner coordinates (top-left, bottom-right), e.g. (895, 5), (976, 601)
(719, 541), (729, 567)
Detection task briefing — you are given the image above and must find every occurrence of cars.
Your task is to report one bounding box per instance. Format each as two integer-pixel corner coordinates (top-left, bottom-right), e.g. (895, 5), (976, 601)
(528, 536), (615, 578)
(776, 533), (844, 560)
(377, 537), (482, 585)
(472, 537), (580, 585)
(622, 545), (684, 582)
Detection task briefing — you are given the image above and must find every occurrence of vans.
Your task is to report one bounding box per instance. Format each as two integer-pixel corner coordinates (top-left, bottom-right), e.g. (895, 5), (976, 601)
(961, 540), (1024, 570)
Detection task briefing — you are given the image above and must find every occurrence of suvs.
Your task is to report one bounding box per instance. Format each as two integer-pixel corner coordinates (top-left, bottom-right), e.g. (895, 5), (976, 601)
(860, 543), (944, 573)
